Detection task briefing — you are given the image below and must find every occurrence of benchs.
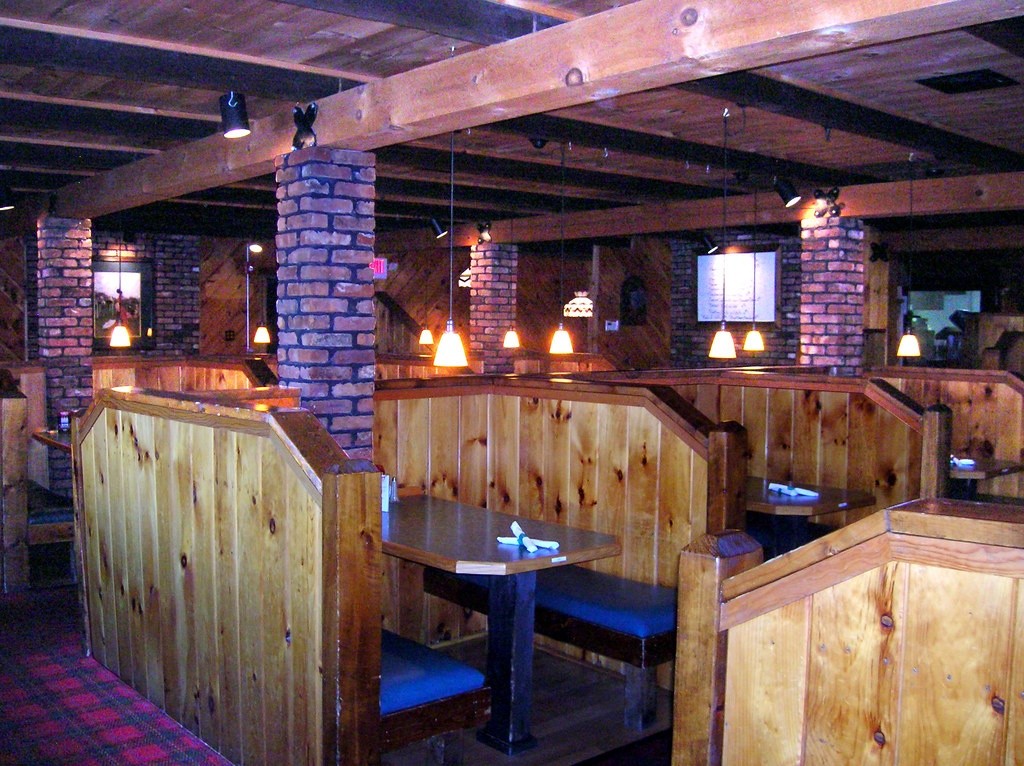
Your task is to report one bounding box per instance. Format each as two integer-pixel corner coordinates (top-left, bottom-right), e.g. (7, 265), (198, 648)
(26, 479), (74, 546)
(379, 627), (493, 766)
(423, 565), (677, 731)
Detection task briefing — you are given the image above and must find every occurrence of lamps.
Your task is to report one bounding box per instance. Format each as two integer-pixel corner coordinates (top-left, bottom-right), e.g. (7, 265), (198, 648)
(743, 180), (765, 351)
(708, 107), (736, 359)
(220, 93), (251, 139)
(502, 216), (520, 349)
(563, 290), (594, 318)
(774, 173), (802, 208)
(548, 141), (574, 356)
(895, 152), (920, 356)
(418, 227), (435, 345)
(254, 321), (271, 343)
(110, 238), (130, 348)
(246, 241), (263, 352)
(432, 132), (467, 367)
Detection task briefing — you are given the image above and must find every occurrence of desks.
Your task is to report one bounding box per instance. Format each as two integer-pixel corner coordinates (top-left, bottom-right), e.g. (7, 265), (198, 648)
(33, 431), (71, 454)
(948, 458), (1024, 498)
(381, 496), (622, 755)
(743, 475), (877, 556)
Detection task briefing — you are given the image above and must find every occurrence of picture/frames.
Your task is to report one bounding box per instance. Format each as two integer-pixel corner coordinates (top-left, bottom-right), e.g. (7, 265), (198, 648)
(92, 261), (155, 349)
